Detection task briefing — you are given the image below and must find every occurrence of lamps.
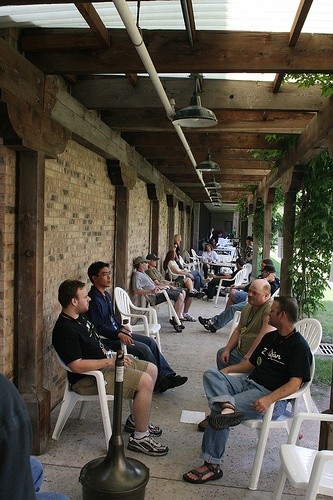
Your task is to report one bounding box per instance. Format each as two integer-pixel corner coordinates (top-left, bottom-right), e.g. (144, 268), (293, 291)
(195, 148), (221, 171)
(170, 77), (218, 128)
(204, 174), (222, 208)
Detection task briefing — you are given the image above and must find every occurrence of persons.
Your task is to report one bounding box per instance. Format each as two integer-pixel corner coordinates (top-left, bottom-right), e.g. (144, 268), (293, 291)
(197, 265), (281, 333)
(85, 261), (188, 393)
(51, 279), (169, 456)
(182, 296), (312, 484)
(0, 373), (70, 500)
(226, 259), (274, 309)
(217, 279), (277, 375)
(163, 226), (254, 301)
(132, 254), (196, 332)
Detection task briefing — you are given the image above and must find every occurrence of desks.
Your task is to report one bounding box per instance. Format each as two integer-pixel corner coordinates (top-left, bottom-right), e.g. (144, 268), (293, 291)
(212, 247), (236, 258)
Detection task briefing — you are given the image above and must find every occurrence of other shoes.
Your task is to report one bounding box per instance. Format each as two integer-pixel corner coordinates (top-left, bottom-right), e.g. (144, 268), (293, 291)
(189, 289), (205, 299)
(179, 317), (187, 321)
(157, 375), (188, 394)
(198, 415), (210, 431)
(170, 316), (185, 333)
(183, 313), (196, 322)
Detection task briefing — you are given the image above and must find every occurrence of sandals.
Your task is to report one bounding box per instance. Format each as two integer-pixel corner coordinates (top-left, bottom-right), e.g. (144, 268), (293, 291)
(183, 462), (223, 484)
(208, 405), (245, 431)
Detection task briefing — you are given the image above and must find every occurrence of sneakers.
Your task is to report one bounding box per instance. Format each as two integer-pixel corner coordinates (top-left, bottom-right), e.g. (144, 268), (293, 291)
(127, 433), (169, 456)
(198, 316), (217, 333)
(124, 413), (162, 437)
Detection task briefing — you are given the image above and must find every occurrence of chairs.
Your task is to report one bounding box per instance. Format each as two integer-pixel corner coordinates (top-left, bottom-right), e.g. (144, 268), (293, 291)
(50, 249), (333, 500)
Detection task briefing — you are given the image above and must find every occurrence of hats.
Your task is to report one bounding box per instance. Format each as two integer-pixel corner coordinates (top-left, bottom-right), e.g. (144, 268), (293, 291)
(133, 256), (150, 265)
(146, 253), (160, 260)
(259, 265), (276, 273)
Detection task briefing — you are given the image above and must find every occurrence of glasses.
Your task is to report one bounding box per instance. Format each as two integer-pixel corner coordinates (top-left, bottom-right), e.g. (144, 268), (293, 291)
(97, 271), (112, 276)
